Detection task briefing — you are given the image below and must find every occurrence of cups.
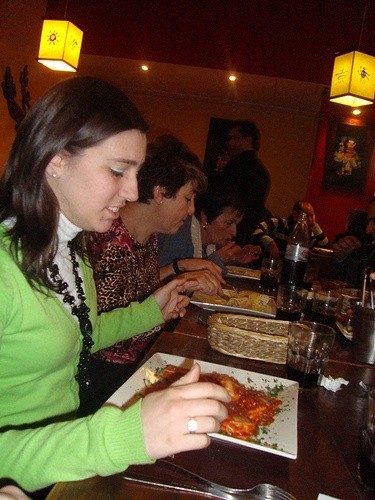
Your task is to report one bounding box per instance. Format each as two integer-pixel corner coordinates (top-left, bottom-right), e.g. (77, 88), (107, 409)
(310, 290), (343, 326)
(358, 426), (375, 490)
(276, 284), (308, 322)
(258, 259), (283, 292)
(286, 321), (336, 392)
(349, 298), (375, 365)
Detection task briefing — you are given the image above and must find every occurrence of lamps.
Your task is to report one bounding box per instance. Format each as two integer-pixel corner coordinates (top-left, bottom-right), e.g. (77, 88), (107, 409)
(36, 0), (84, 73)
(324, 118), (369, 197)
(328, 0), (375, 108)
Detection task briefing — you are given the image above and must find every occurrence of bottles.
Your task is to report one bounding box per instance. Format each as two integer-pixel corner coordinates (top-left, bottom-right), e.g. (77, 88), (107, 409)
(282, 209), (311, 286)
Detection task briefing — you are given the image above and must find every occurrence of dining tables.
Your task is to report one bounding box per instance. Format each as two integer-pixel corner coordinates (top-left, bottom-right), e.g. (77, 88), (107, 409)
(174, 240), (375, 365)
(53, 332), (375, 500)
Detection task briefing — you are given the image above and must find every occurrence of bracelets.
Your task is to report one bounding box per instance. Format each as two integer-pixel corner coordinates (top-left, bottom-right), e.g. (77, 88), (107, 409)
(172, 257), (182, 275)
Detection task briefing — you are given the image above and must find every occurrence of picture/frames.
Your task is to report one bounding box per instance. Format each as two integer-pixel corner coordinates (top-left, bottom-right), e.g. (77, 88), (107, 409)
(204, 116), (235, 176)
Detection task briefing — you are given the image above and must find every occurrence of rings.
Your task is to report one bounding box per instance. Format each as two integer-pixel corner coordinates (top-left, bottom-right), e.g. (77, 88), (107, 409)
(187, 417), (198, 434)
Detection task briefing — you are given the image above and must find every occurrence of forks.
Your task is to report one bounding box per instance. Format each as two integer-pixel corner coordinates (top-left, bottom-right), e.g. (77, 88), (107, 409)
(159, 459), (297, 500)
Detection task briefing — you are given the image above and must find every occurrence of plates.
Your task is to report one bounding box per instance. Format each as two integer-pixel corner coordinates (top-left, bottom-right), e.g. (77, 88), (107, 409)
(104, 351), (300, 459)
(225, 265), (260, 281)
(190, 289), (277, 318)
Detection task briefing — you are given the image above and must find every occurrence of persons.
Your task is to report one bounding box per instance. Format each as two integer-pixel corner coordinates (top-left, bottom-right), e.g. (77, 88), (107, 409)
(156, 190), (262, 275)
(331, 196), (375, 290)
(249, 200), (329, 262)
(201, 118), (270, 247)
(73, 132), (228, 388)
(0, 74), (232, 493)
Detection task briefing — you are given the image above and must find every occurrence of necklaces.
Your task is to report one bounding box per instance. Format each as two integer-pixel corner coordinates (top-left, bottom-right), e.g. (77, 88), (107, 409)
(42, 241), (97, 392)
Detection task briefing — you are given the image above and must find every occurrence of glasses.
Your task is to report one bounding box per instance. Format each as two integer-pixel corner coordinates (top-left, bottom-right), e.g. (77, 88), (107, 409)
(225, 135), (250, 143)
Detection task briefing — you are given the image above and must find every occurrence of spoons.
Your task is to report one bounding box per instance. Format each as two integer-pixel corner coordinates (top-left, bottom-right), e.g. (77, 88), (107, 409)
(122, 469), (255, 500)
